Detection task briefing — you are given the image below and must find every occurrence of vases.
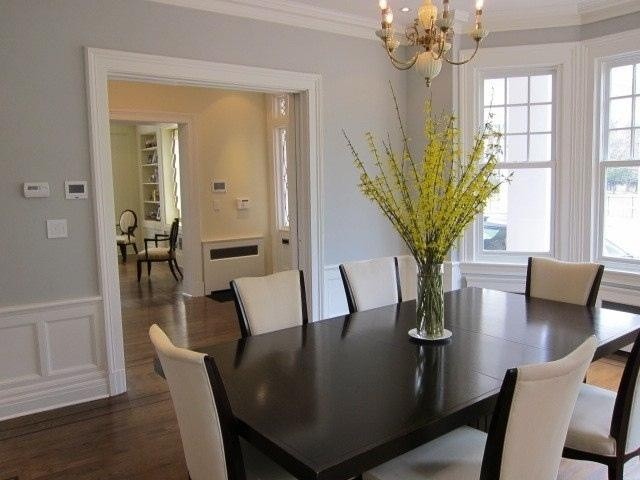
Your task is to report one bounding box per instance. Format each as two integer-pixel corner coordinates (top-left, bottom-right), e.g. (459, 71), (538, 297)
(408, 265), (454, 343)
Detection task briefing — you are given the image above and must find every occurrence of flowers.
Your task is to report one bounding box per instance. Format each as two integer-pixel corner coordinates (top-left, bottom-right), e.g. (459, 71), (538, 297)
(342, 80), (515, 335)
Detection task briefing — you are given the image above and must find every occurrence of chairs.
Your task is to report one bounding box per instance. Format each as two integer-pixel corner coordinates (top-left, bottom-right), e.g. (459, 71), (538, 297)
(116, 209), (138, 265)
(136, 217), (184, 282)
(339, 258), (397, 311)
(562, 327), (640, 480)
(229, 268), (307, 335)
(358, 335), (601, 479)
(397, 253), (457, 302)
(147, 322), (249, 478)
(525, 256), (606, 310)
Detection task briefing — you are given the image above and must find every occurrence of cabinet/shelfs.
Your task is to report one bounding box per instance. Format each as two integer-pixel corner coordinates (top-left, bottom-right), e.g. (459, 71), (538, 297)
(135, 124), (175, 230)
(201, 235), (263, 296)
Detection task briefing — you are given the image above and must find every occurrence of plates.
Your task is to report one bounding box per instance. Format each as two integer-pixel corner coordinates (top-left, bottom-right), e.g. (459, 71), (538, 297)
(408, 328), (453, 341)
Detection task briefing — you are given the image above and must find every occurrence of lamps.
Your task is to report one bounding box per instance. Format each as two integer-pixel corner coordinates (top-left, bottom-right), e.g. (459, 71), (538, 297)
(377, 0), (490, 88)
